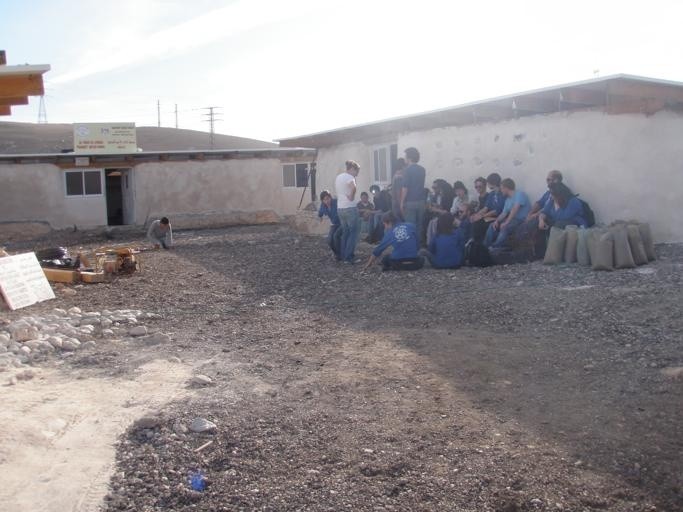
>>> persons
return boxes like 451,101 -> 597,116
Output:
316,169 -> 594,272
334,159 -> 361,265
398,148 -> 428,255
145,216 -> 172,250
390,157 -> 405,224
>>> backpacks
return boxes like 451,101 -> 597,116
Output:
580,200 -> 595,228
